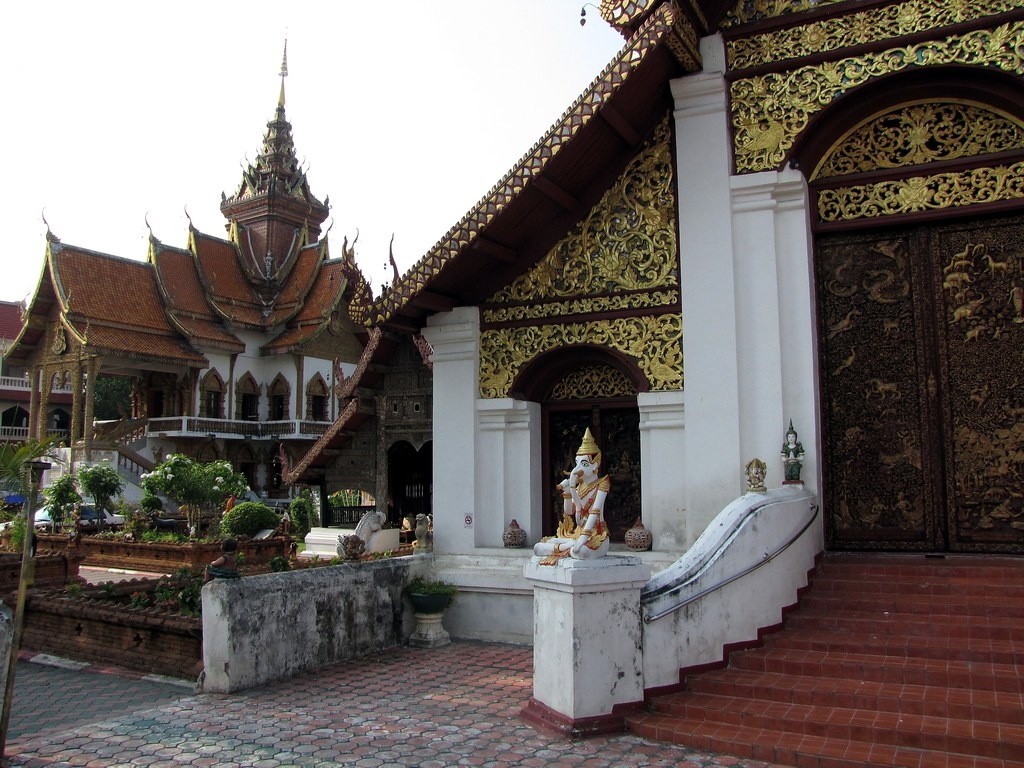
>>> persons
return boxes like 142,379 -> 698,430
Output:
780,418 -> 805,479
746,463 -> 765,488
534,429 -> 610,559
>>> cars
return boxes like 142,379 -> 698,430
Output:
0,501 -> 129,536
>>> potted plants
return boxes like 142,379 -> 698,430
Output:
402,575 -> 458,613
